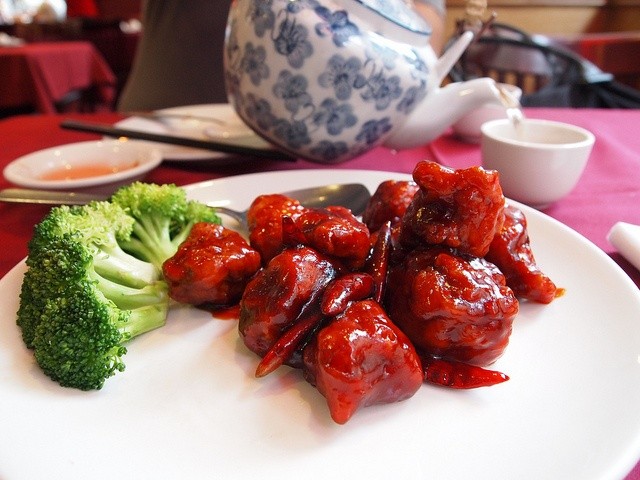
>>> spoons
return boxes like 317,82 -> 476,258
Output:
0,182 -> 370,229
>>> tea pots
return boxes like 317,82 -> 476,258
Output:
223,0 -> 512,163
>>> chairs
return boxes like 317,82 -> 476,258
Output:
440,37 -> 588,114
455,19 -> 533,49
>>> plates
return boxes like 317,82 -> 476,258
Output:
0,168 -> 640,480
116,103 -> 273,161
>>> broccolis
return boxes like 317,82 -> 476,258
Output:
24,198 -> 159,286
110,181 -> 221,273
16,237 -> 166,390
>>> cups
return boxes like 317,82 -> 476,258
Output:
481,116 -> 595,212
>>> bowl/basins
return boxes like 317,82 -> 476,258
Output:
4,138 -> 161,187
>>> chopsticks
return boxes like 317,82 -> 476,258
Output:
57,121 -> 296,162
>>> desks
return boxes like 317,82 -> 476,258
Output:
0,40 -> 117,114
